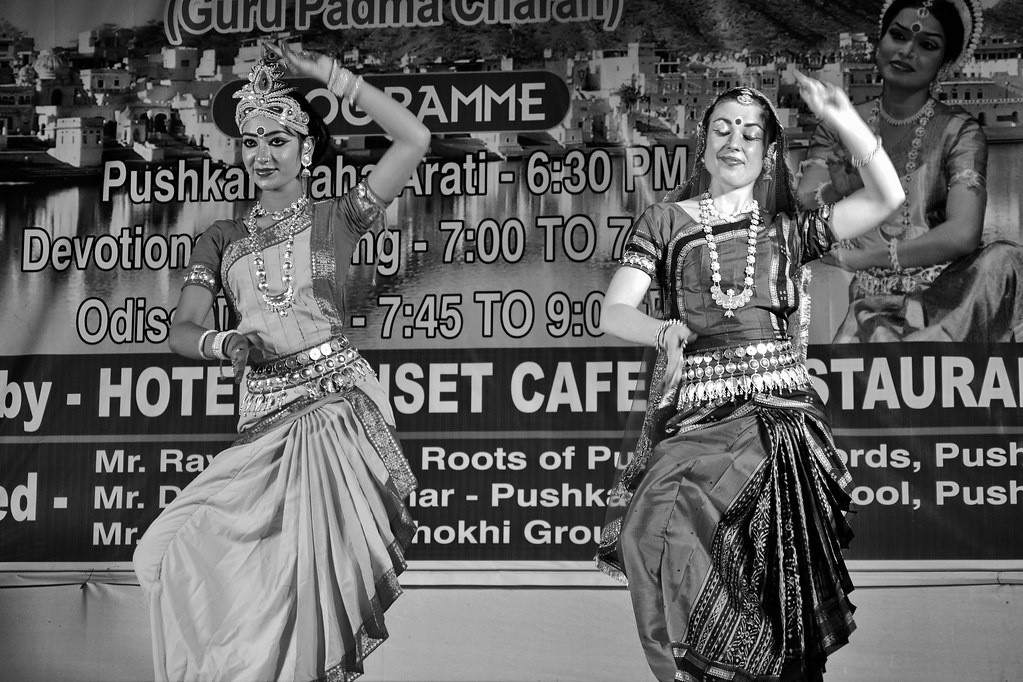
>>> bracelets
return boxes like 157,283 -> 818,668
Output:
889,236 -> 903,272
655,319 -> 691,356
198,329 -> 244,366
852,135 -> 883,169
328,59 -> 363,107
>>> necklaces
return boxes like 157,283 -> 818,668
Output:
248,194 -> 308,317
868,97 -> 934,229
699,191 -> 759,318
879,99 -> 931,125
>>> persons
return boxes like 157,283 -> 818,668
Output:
133,42 -> 431,682
795,0 -> 1023,343
595,69 -> 907,682
